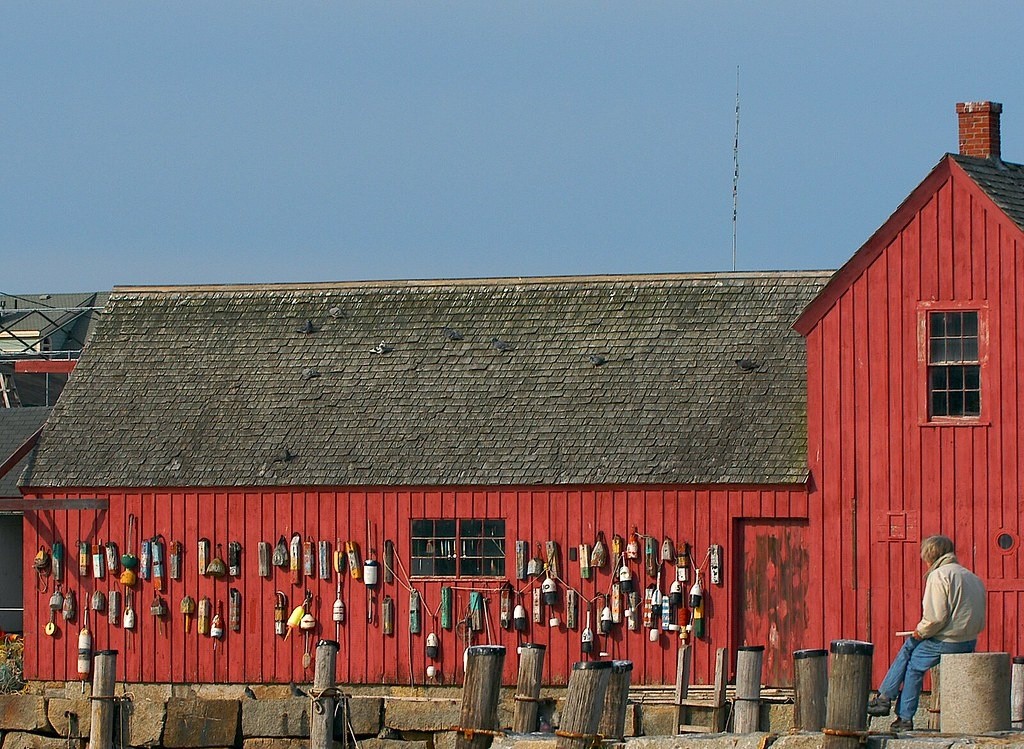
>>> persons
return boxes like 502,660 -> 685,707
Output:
867,535 -> 987,729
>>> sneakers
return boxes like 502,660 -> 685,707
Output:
868,697 -> 891,717
890,716 -> 913,732
